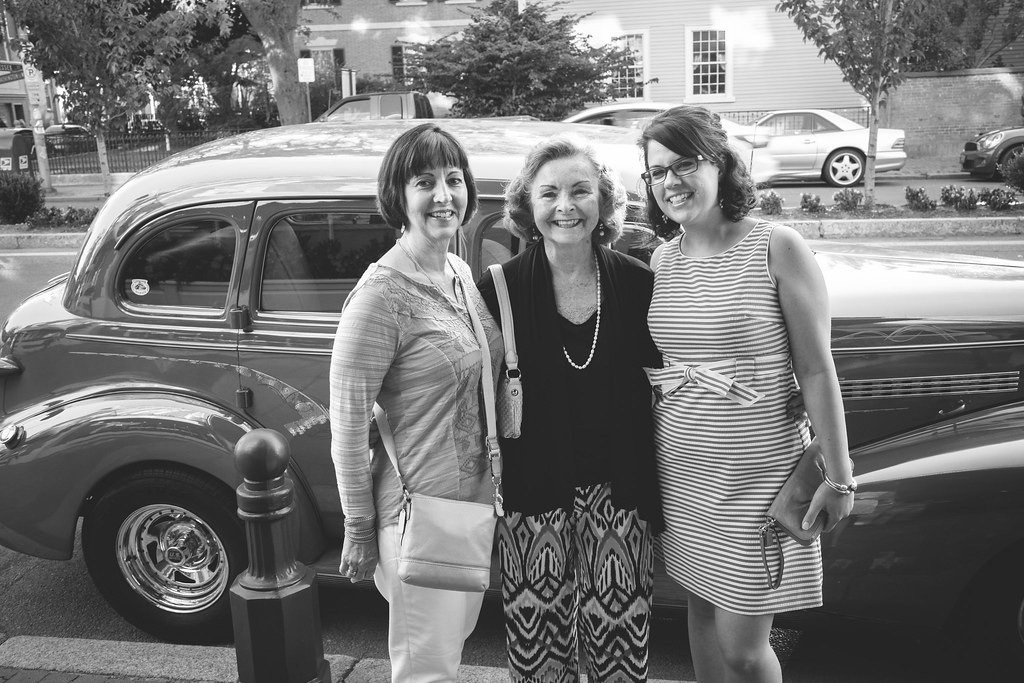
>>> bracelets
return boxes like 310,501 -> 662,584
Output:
344,527 -> 376,543
824,472 -> 857,495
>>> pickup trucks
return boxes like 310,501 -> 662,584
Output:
311,89 -> 542,123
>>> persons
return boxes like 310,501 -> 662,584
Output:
642,105 -> 858,683
329,123 -> 504,683
476,138 -> 665,683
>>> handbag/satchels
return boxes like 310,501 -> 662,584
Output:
487,263 -> 523,438
398,493 -> 498,593
758,436 -> 855,590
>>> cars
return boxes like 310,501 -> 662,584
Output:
552,100 -> 779,192
958,125 -> 1024,185
0,114 -> 1024,683
1,119 -> 171,162
739,108 -> 908,188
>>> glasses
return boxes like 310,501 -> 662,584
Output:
642,153 -> 714,186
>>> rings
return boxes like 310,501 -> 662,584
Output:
347,569 -> 357,575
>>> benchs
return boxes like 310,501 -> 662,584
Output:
143,220 -> 320,311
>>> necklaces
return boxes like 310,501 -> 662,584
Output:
397,239 -> 483,347
562,249 -> 601,370
